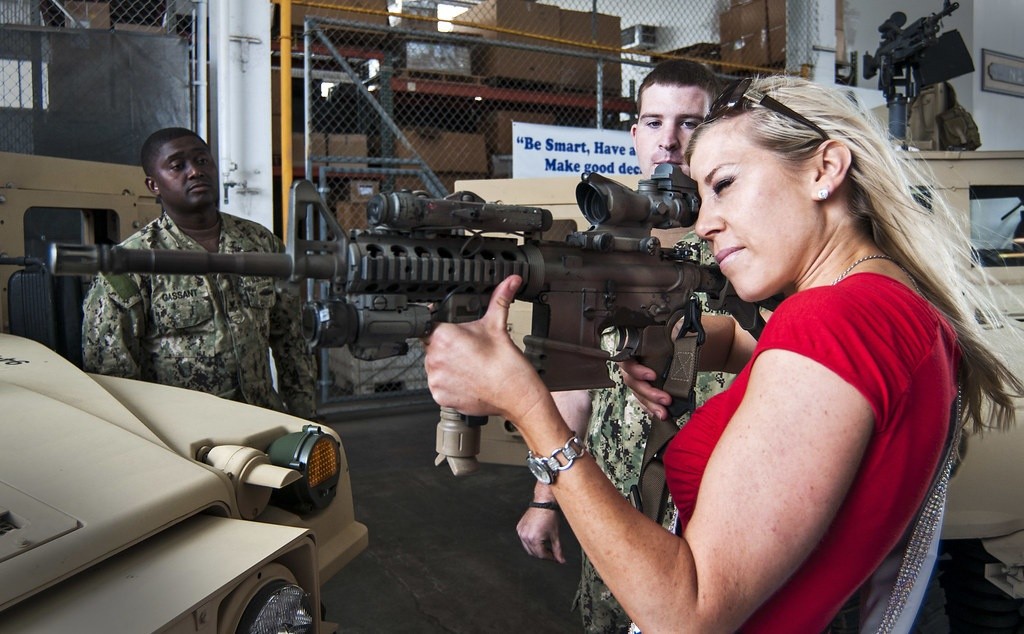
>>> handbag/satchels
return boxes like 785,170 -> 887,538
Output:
936,105 -> 981,152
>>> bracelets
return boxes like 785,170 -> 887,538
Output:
526,498 -> 561,512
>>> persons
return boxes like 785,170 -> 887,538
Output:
82,128 -> 322,428
516,58 -> 779,634
424,75 -> 1024,634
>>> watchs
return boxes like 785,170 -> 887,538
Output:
525,430 -> 586,485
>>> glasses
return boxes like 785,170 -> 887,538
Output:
703,77 -> 830,141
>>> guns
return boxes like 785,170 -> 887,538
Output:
862,0 -> 961,100
46,164 -> 784,421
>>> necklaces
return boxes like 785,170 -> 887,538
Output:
828,254 -> 938,298
